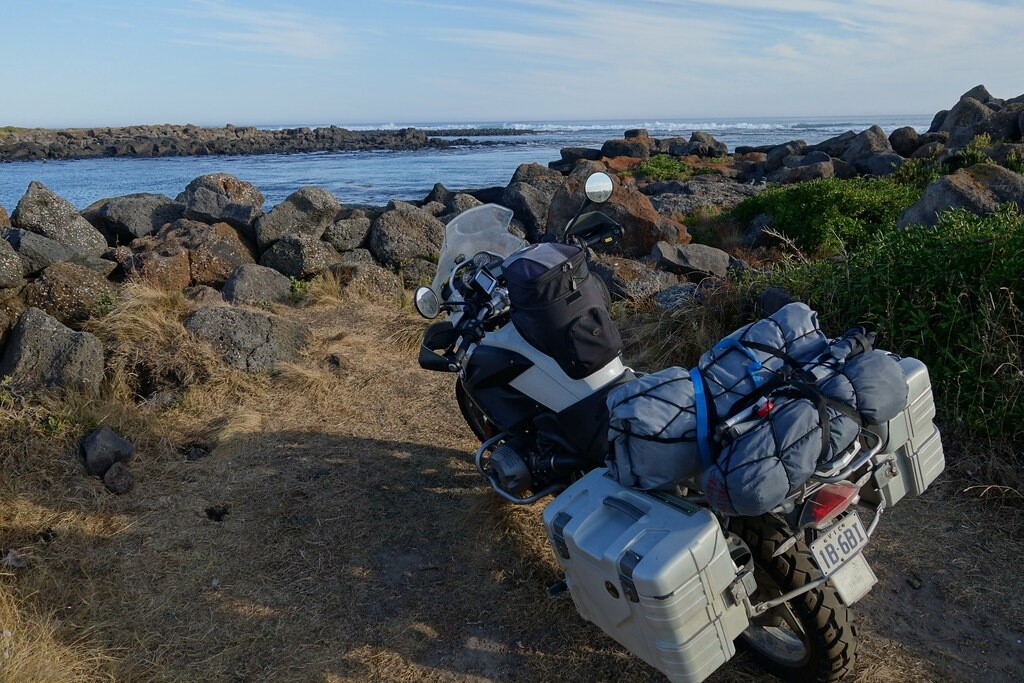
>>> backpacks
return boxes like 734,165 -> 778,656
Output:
503,242 -> 623,380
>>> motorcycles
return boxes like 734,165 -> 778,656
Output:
415,172 -> 946,683
